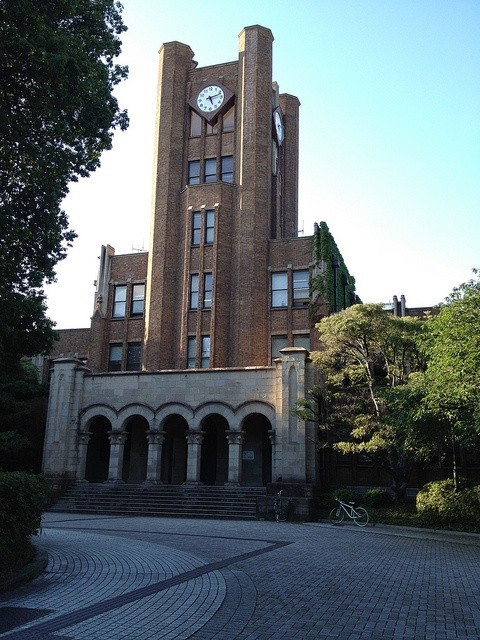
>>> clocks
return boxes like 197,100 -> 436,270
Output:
272,105 -> 287,149
188,78 -> 237,124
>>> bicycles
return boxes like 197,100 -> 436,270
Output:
329,495 -> 369,527
271,489 -> 288,523
